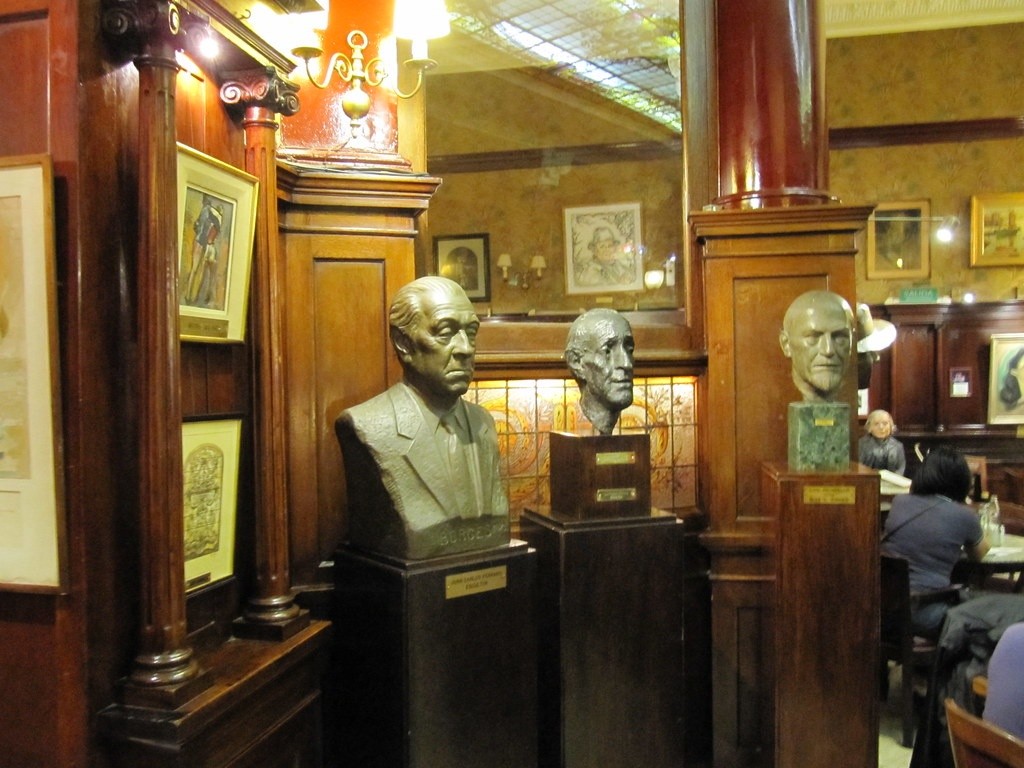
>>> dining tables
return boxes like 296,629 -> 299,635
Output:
954,534 -> 1024,594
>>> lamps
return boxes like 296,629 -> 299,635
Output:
497,254 -> 546,289
290,0 -> 450,138
644,269 -> 665,291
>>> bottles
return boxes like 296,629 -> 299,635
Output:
986,494 -> 1002,548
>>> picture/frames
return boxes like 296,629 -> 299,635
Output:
986,333 -> 1024,424
562,201 -> 645,296
949,367 -> 972,398
1,153 -> 69,595
176,141 -> 260,343
432,233 -> 491,303
182,411 -> 246,599
865,197 -> 932,280
970,191 -> 1024,266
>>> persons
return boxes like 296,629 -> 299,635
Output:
333,276 -> 510,560
777,290 -> 855,404
857,408 -> 907,476
562,308 -> 634,436
981,623 -> 1024,739
879,447 -> 993,716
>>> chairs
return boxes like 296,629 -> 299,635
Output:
880,555 -> 937,747
945,698 -> 1024,768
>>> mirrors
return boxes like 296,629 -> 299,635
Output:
425,0 -> 688,327
